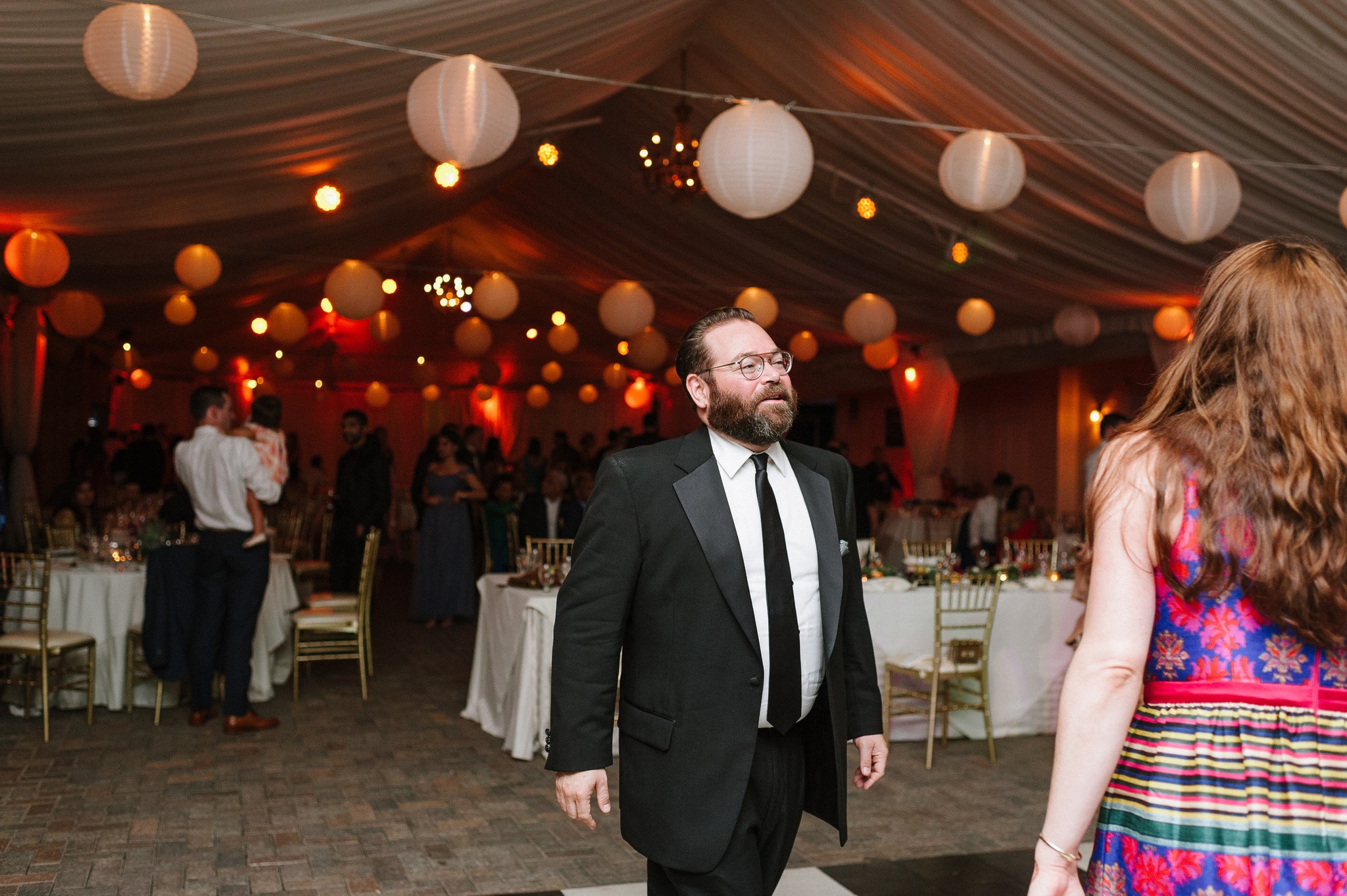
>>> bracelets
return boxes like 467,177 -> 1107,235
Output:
1038,832 -> 1083,864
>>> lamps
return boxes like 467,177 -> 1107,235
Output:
638,45 -> 708,202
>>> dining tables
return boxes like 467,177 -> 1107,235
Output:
3,551 -> 304,710
457,568 -> 1087,768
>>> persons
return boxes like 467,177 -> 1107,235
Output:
108,412 -> 1134,571
416,428 -> 488,630
172,384 -> 282,731
52,474 -> 95,546
232,395 -> 289,546
549,307 -> 886,894
1026,236 -> 1347,894
327,408 -> 391,626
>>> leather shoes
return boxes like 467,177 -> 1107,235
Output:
191,706 -> 219,726
224,710 -> 281,732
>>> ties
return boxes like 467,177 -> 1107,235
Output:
750,451 -> 803,736
996,504 -> 1006,540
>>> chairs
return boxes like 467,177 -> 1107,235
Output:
21,484 -> 171,557
0,551 -> 97,743
901,537 -> 953,559
881,570 -> 1007,769
1002,536 -> 1060,573
123,622 -> 226,726
525,535 -> 576,568
480,510 -> 523,578
258,499 -> 383,702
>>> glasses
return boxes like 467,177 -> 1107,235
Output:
693,350 -> 796,384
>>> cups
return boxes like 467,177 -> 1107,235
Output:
520,548 -> 537,573
